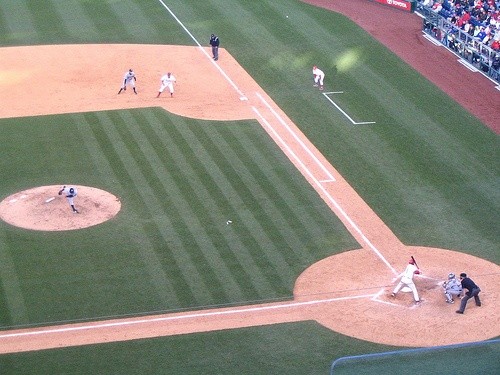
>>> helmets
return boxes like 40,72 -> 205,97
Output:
448,272 -> 454,278
70,188 -> 74,193
408,258 -> 414,264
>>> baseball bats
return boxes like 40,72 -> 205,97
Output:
411,255 -> 419,269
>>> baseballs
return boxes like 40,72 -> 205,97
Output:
227,221 -> 232,224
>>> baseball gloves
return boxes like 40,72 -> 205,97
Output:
442,281 -> 446,288
58,190 -> 62,195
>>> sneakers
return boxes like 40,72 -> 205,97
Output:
445,299 -> 454,303
456,294 -> 463,298
391,293 -> 395,297
415,301 -> 419,305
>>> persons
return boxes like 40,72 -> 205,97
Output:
156,73 -> 176,97
58,186 -> 80,214
456,273 -> 481,314
417,0 -> 500,83
209,34 -> 220,61
440,273 -> 463,303
390,258 -> 423,304
312,65 -> 325,90
117,69 -> 138,94
